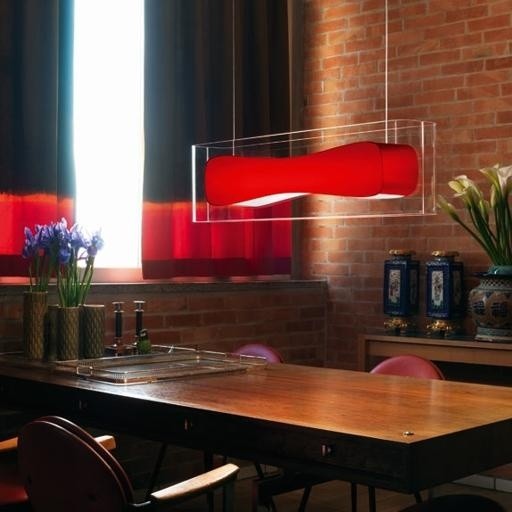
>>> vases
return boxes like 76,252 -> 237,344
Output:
24,289 -> 106,360
464,266 -> 511,341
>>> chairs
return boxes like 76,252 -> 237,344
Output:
233,343 -> 447,512
17,415 -> 241,511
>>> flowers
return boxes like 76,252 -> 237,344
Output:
436,162 -> 512,267
22,217 -> 105,307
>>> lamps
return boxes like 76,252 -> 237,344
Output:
190,1 -> 437,224
